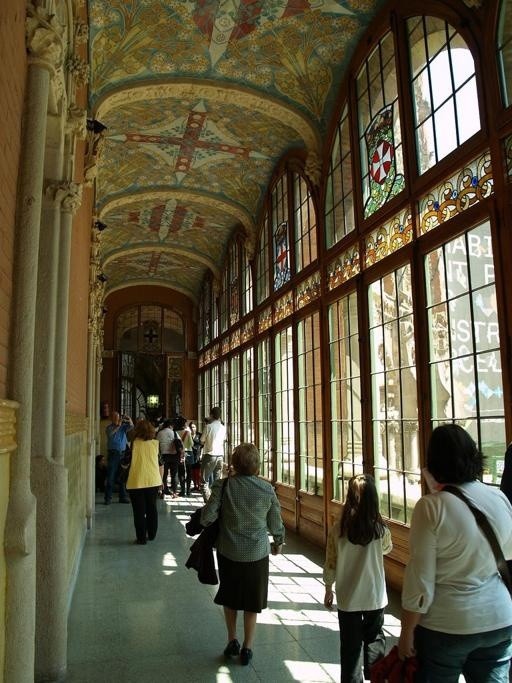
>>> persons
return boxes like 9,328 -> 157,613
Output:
122,417 -> 164,545
199,441 -> 286,667
322,474 -> 394,682
89,400 -> 227,508
396,422 -> 511,682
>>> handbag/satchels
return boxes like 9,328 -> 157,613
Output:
123,445 -> 132,465
174,439 -> 183,452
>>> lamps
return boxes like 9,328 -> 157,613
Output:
95,222 -> 108,231
86,119 -> 107,134
97,274 -> 109,282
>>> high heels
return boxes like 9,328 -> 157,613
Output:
241,649 -> 253,666
224,639 -> 240,656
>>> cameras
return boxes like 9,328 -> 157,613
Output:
121,415 -> 126,420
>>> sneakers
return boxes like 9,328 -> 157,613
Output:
158,489 -> 190,499
119,498 -> 130,504
137,534 -> 156,544
104,499 -> 112,504
